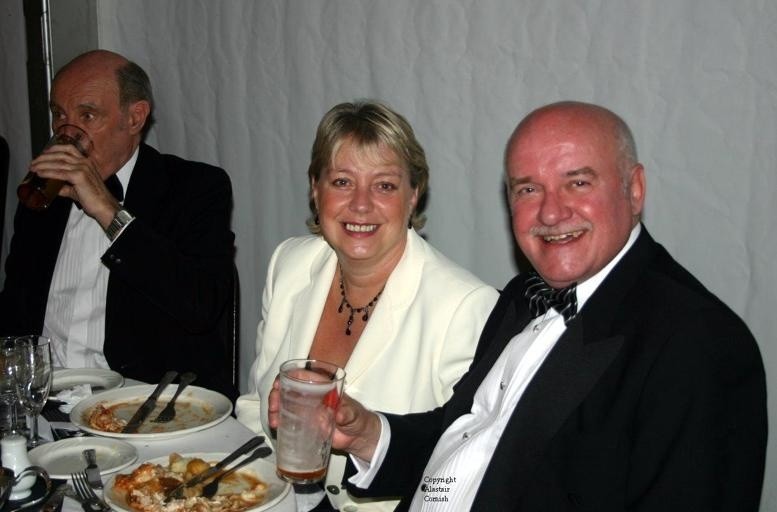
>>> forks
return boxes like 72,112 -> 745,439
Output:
68,470 -> 109,512
149,370 -> 198,422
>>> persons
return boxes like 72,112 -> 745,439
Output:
234,101 -> 500,511
0,49 -> 242,418
268,101 -> 768,512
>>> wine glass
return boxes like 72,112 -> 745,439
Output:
0,335 -> 52,446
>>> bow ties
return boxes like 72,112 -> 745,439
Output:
72,173 -> 123,209
521,269 -> 580,324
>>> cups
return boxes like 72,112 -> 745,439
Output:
16,124 -> 93,213
274,357 -> 348,486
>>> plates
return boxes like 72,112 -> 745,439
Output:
103,451 -> 291,511
48,365 -> 122,397
27,436 -> 139,480
67,383 -> 236,441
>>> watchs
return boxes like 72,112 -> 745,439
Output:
105,207 -> 133,243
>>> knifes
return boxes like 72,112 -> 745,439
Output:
153,436 -> 270,500
81,449 -> 103,490
119,370 -> 178,436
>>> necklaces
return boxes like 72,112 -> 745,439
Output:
338,263 -> 385,335
